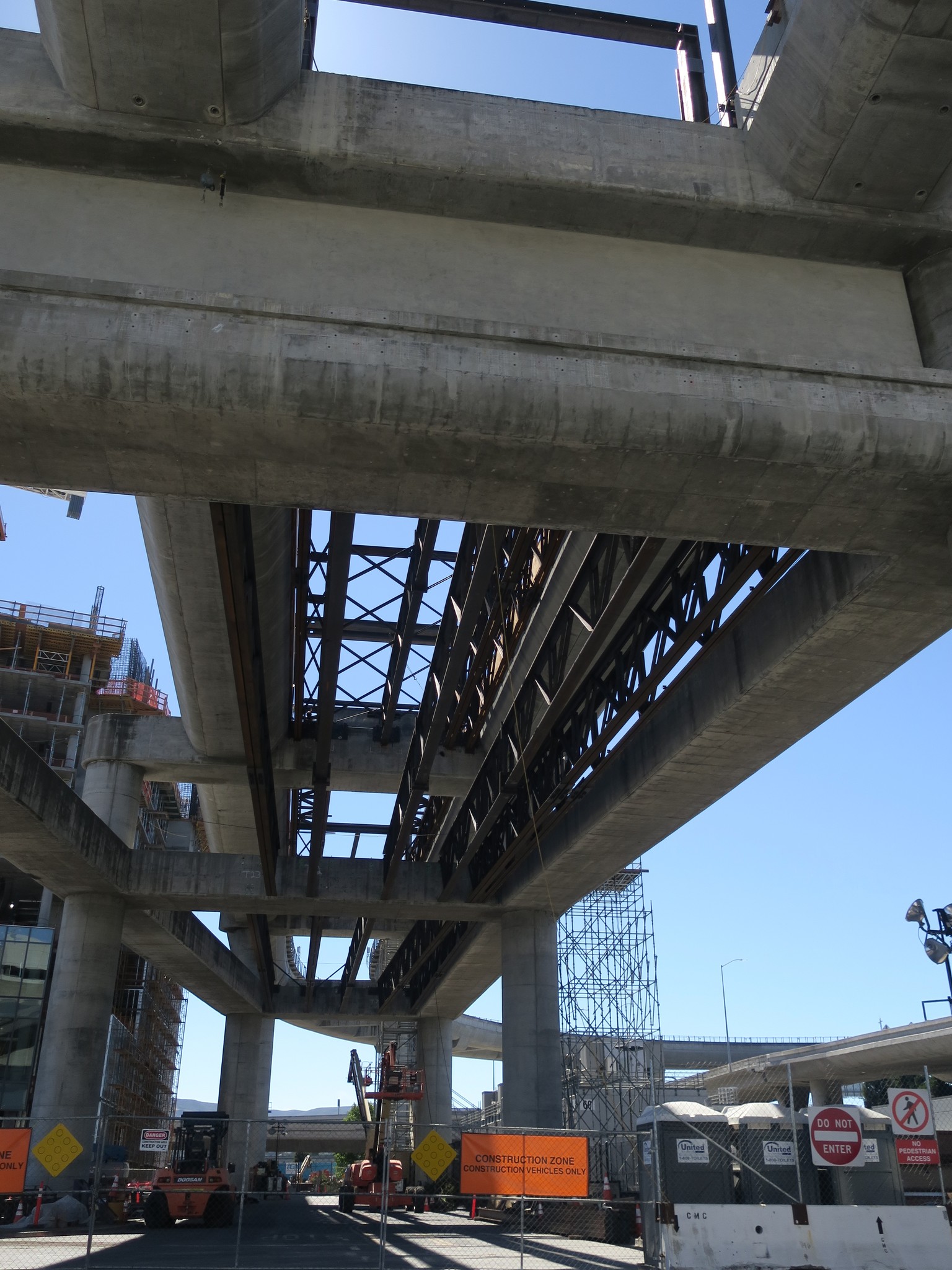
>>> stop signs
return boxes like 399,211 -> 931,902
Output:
808,1107 -> 866,1167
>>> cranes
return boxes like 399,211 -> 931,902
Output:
0,481 -> 87,544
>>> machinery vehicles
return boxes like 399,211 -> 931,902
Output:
142,1111 -> 234,1230
339,1040 -> 427,1213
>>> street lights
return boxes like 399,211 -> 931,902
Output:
904,898 -> 952,995
720,957 -> 742,1072
